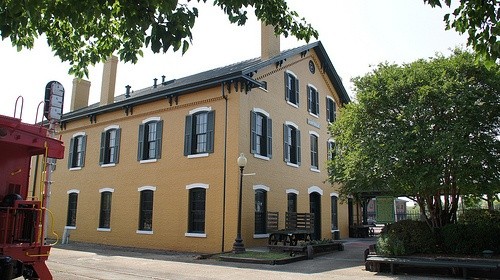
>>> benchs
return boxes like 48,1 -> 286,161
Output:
366,257 -> 500,280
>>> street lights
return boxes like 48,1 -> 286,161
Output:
231,152 -> 247,254
